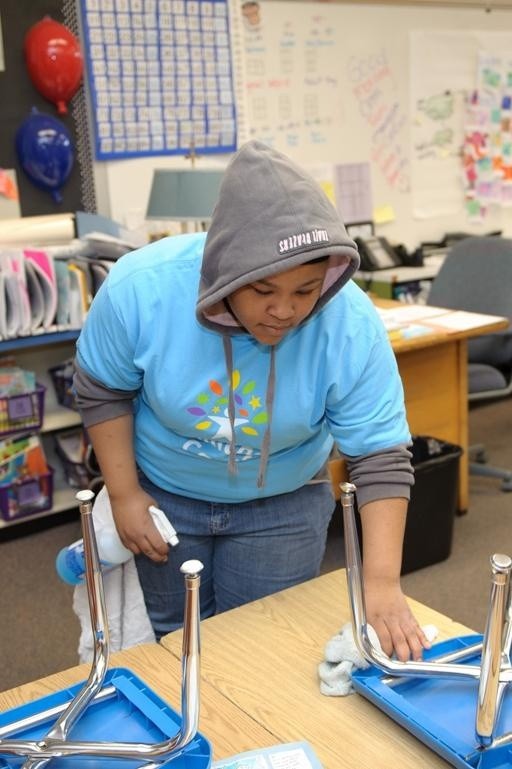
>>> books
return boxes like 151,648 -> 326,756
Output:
2,234 -> 142,338
0,356 -> 40,431
0,432 -> 50,517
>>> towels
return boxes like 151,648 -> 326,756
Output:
71,479 -> 157,662
317,622 -> 437,697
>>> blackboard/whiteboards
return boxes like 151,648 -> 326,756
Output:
71,2 -> 511,255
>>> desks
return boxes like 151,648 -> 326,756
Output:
160,564 -> 510,769
349,255 -> 437,303
1,640 -> 320,769
327,288 -> 511,523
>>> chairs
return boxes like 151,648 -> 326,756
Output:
427,237 -> 512,494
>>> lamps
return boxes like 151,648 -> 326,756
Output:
144,165 -> 222,237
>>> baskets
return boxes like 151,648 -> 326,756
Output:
55,430 -> 97,489
48,363 -> 80,412
0,458 -> 56,521
1,381 -> 47,436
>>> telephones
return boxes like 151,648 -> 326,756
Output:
355,235 -> 400,271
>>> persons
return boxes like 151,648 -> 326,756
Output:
73,140 -> 431,666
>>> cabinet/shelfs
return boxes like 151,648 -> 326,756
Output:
0,254 -> 114,529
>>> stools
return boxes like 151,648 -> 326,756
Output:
1,487 -> 211,768
336,471 -> 511,769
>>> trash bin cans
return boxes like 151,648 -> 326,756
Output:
402,435 -> 464,577
